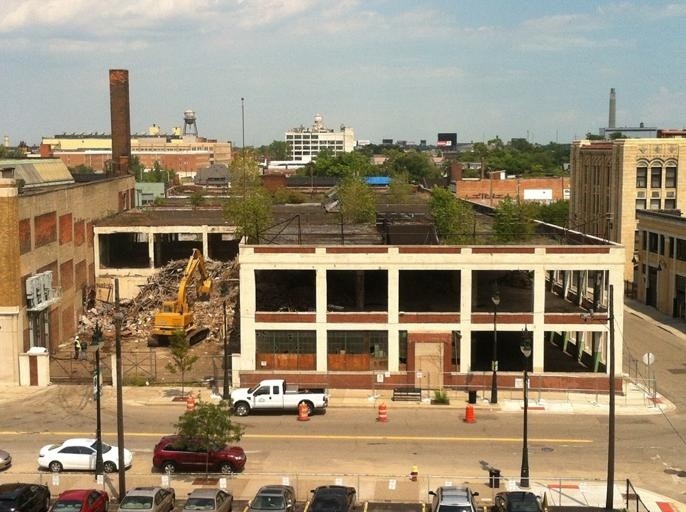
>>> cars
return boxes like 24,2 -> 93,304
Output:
522,504 -> 527,506
117,486 -> 175,512
245,484 -> 297,512
494,492 -> 543,512
0,483 -> 51,512
181,488 -> 232,512
429,486 -> 479,512
0,449 -> 11,471
49,489 -> 109,512
305,485 -> 356,512
37,438 -> 132,474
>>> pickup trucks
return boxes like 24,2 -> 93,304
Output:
230,379 -> 328,417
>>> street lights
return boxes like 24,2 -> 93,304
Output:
88,321 -> 104,480
488,291 -> 501,402
515,319 -> 533,485
221,300 -> 236,402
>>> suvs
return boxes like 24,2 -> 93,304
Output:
152,434 -> 246,475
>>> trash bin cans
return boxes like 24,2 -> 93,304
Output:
468,390 -> 477,404
490,469 -> 501,488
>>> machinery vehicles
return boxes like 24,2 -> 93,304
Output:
145,247 -> 214,347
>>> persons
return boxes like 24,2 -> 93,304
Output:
73,336 -> 81,360
